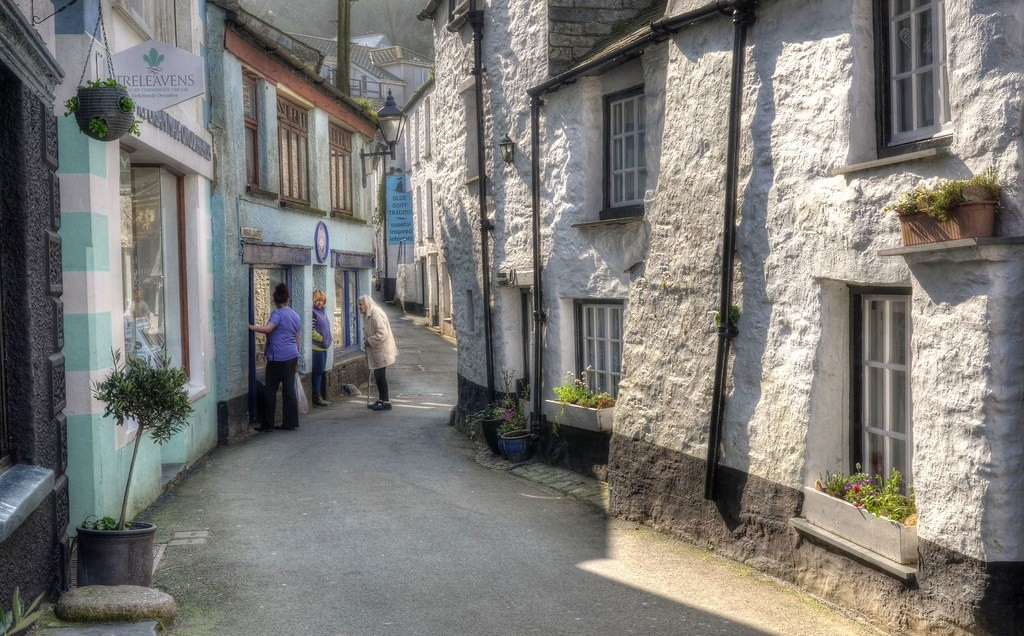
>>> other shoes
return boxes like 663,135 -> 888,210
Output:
314,395 -> 332,406
274,424 -> 295,430
254,426 -> 273,432
368,401 -> 392,410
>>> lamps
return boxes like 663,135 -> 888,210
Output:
497,134 -> 516,165
375,87 -> 408,160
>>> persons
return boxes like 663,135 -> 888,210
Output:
312,289 -> 333,402
357,294 -> 399,410
248,283 -> 302,430
128,287 -> 150,321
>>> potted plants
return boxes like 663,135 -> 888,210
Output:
501,379 -> 536,464
62,78 -> 143,143
882,172 -> 1002,248
75,343 -> 194,588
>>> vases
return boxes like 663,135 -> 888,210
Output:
545,399 -> 616,433
481,417 -> 508,456
804,485 -> 919,566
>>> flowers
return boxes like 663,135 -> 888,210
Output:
814,464 -> 919,528
465,368 -> 517,439
552,365 -> 617,439
